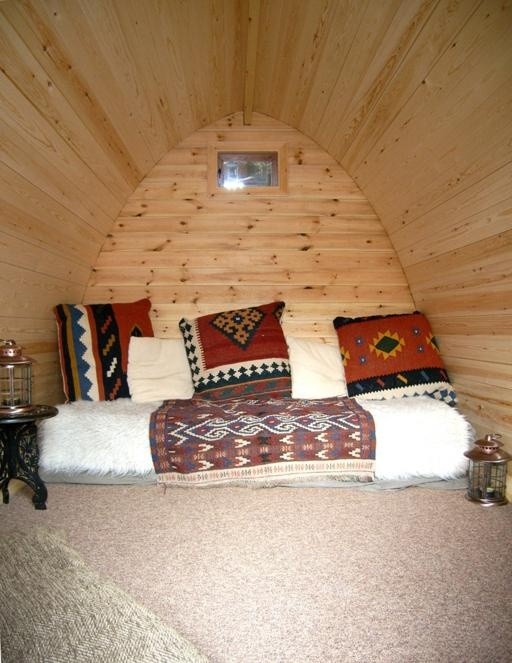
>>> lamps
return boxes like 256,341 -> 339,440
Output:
464,433 -> 511,506
0,339 -> 36,416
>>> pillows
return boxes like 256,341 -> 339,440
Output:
179,300 -> 293,400
287,338 -> 350,399
127,336 -> 197,402
55,298 -> 154,402
333,310 -> 456,403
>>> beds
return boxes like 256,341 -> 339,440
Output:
34,398 -> 474,489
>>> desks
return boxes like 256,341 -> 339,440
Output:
0,405 -> 59,511
